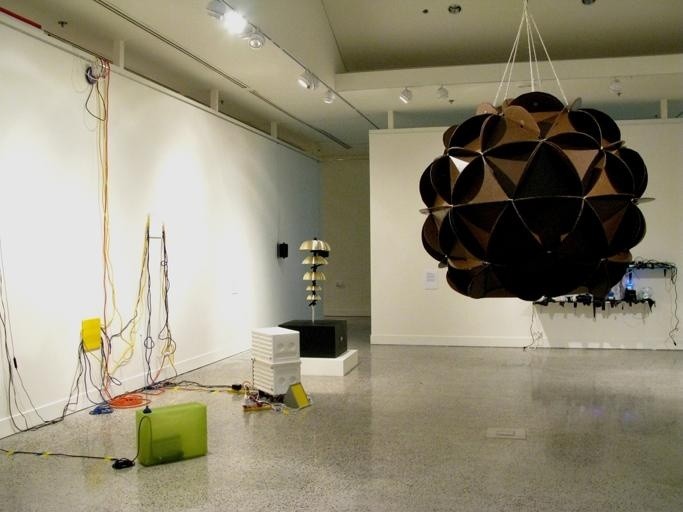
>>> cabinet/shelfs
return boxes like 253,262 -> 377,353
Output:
535,266 -> 674,318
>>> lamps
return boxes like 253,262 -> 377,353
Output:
206,0 -> 334,104
400,87 -> 413,103
436,85 -> 448,100
